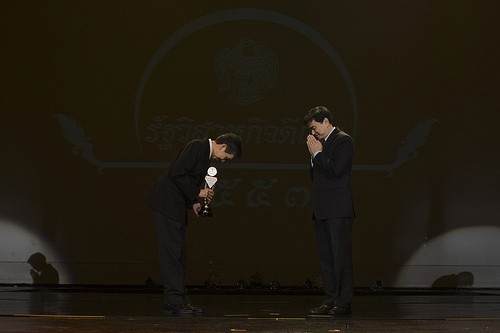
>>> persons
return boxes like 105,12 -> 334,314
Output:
304,106 -> 356,315
144,133 -> 242,314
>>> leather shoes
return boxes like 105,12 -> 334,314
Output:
310,305 -> 329,314
328,304 -> 352,315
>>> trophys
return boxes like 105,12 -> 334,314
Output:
197,167 -> 217,216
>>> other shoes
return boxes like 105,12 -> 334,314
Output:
184,305 -> 205,313
165,305 -> 195,314
